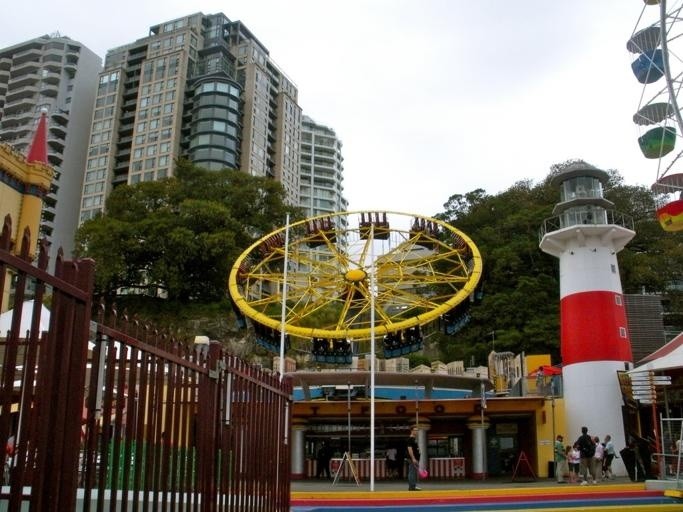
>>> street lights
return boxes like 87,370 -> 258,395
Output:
554,446 -> 565,453
419,468 -> 429,479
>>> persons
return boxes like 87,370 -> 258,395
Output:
385,445 -> 397,475
549,378 -> 554,396
314,441 -> 333,480
551,373 -> 559,396
675,439 -> 683,455
662,430 -> 676,477
534,365 -> 544,396
406,425 -> 420,491
552,426 -> 620,486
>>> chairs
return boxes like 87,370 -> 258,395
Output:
409,486 -> 421,491
557,475 -> 614,485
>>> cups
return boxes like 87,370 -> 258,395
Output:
413,430 -> 419,431
